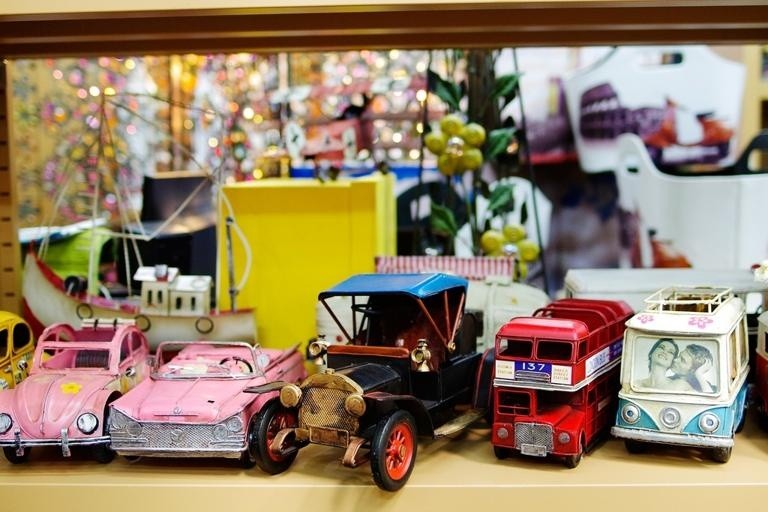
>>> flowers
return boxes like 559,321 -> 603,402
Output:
407,48 -> 539,283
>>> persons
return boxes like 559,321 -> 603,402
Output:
634,338 -> 718,394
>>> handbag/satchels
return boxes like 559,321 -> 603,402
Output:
562,45 -> 745,175
